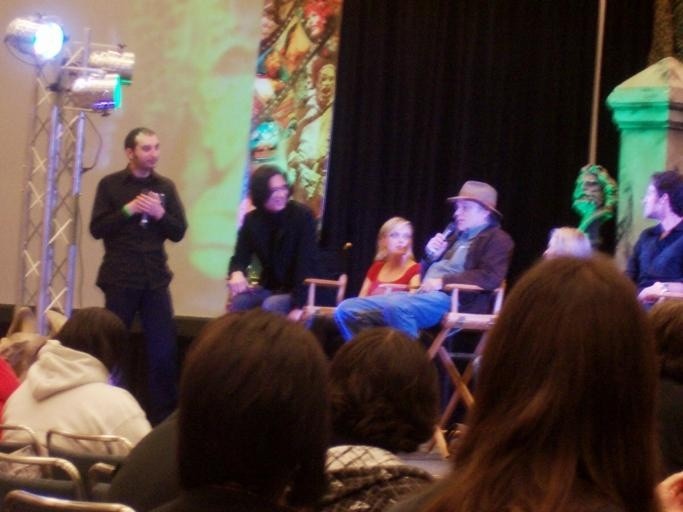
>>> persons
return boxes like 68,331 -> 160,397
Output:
624,169 -> 683,292
227,165 -> 319,315
90,128 -> 187,423
562,162 -> 618,254
544,225 -> 595,261
333,179 -> 515,343
238,1 -> 341,225
2,255 -> 682,511
358,217 -> 422,299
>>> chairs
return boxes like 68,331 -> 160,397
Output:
226,273 -> 348,320
378,280 -> 506,458
616,264 -> 682,310
0,425 -> 136,512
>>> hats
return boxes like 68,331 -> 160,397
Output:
448,180 -> 503,216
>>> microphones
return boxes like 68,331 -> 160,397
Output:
425,222 -> 457,260
138,187 -> 153,227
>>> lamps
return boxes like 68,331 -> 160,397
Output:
3,14 -> 136,117
